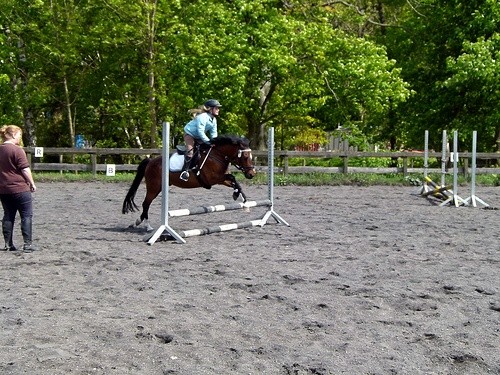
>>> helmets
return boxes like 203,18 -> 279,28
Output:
204,99 -> 223,107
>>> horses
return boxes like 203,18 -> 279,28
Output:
120,132 -> 256,232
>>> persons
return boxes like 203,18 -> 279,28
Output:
0,125 -> 38,251
182,99 -> 222,179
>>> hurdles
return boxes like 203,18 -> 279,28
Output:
410,130 -> 469,208
440,130 -> 490,208
143,121 -> 289,246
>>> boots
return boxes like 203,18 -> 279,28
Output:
21,215 -> 32,252
181,156 -> 193,178
2,216 -> 17,251
